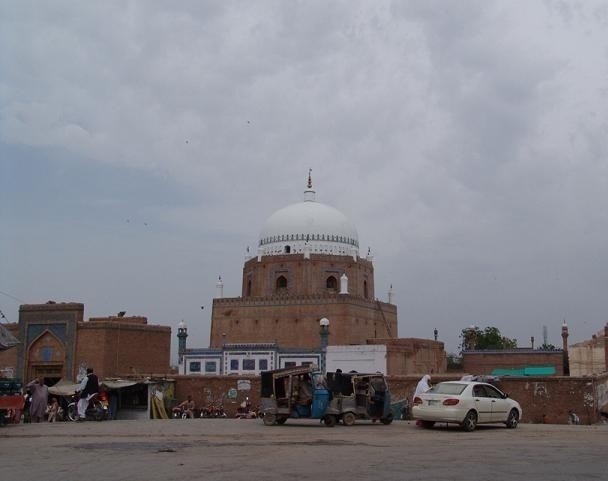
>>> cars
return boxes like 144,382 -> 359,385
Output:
412,381 -> 522,432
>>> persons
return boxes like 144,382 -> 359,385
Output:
172,394 -> 198,420
48,398 -> 59,423
566,410 -> 581,425
25,376 -> 49,424
74,367 -> 99,422
412,374 -> 432,427
358,378 -> 380,417
457,376 -> 476,398
298,373 -> 313,401
542,415 -> 550,424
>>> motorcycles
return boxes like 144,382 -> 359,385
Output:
178,401 -> 226,419
67,390 -> 108,422
259,364 -> 394,427
